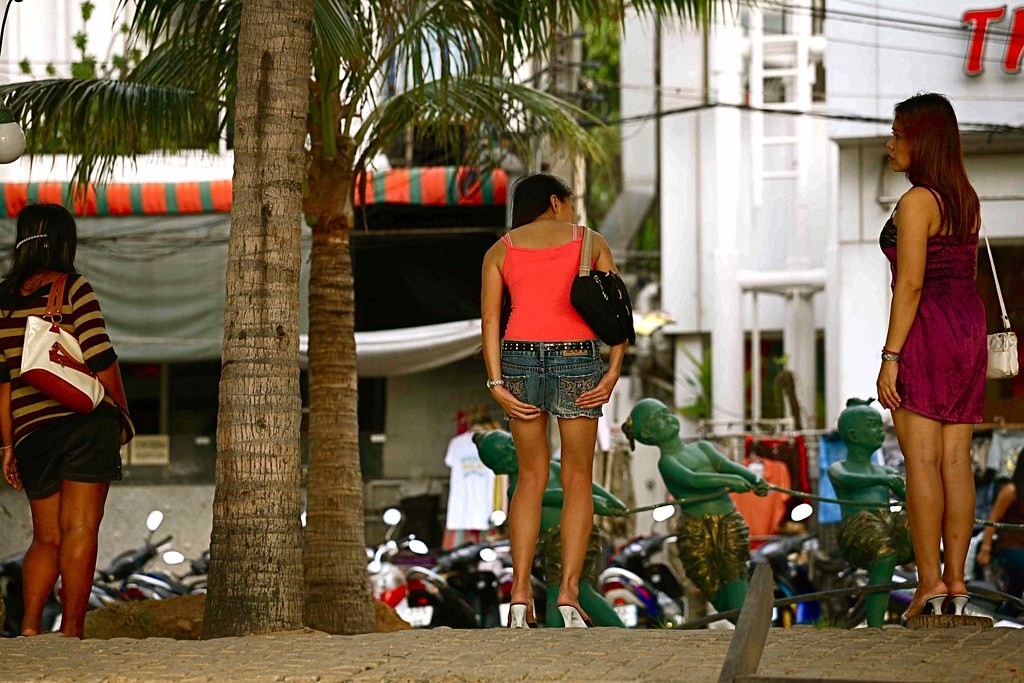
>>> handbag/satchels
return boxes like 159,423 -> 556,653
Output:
570,227 -> 636,346
19,274 -> 105,412
986,332 -> 1019,379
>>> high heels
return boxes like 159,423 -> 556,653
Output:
943,594 -> 970,615
901,593 -> 947,625
558,605 -> 594,628
509,604 -> 538,629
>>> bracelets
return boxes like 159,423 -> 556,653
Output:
981,544 -> 990,552
882,346 -> 900,356
0,444 -> 12,455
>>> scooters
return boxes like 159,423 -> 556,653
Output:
597,505 -> 737,630
364,501 -> 547,635
745,498 -> 1024,628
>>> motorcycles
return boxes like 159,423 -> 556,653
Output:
0,509 -> 212,636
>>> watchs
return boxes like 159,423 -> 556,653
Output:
487,379 -> 505,390
881,353 -> 898,361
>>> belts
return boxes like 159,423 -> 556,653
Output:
503,339 -> 601,352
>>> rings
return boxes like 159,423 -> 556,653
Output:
10,474 -> 14,476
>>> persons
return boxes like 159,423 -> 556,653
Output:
977,447 -> 1024,618
479,175 -> 628,631
621,397 -> 768,627
875,92 -> 987,620
0,202 -> 136,641
475,427 -> 627,629
828,398 -> 914,630
